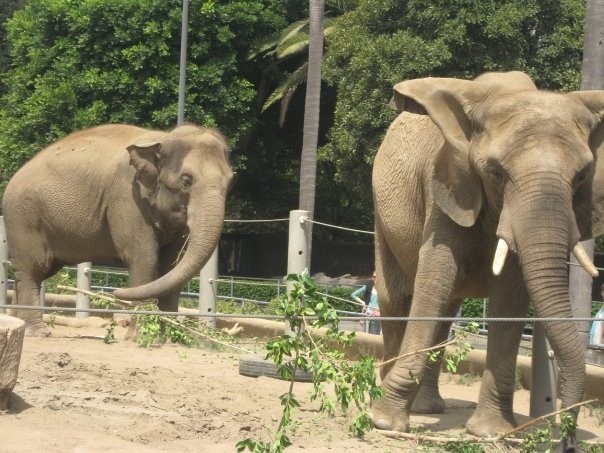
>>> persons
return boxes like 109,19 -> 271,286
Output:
350,268 -> 380,335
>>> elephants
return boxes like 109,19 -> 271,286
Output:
1,123 -> 238,337
366,71 -> 604,453
